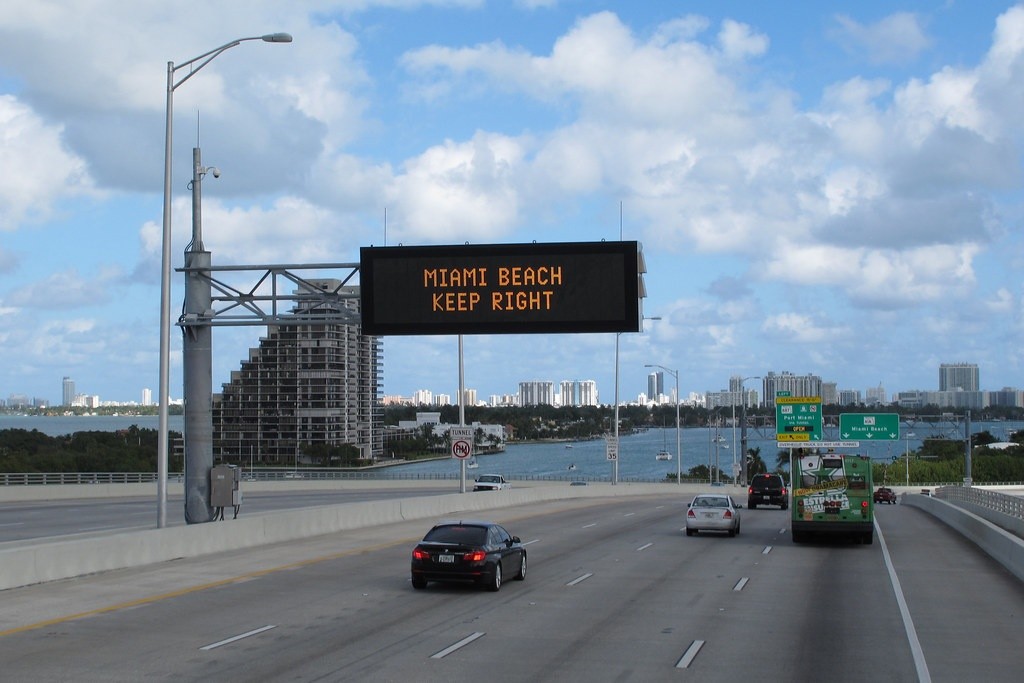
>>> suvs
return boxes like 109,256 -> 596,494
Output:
746,472 -> 791,511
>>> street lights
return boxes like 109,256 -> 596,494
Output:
611,316 -> 661,483
645,364 -> 680,486
732,376 -> 762,487
157,31 -> 294,527
906,431 -> 916,486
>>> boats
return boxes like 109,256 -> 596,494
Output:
467,452 -> 478,469
569,464 -> 576,471
712,432 -> 730,449
565,440 -> 574,449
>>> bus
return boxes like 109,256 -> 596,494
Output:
789,448 -> 874,545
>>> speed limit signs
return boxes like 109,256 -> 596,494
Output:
606,442 -> 618,460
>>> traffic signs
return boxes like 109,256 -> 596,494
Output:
774,396 -> 823,442
838,413 -> 900,442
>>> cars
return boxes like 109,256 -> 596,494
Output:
411,520 -> 527,592
685,493 -> 742,538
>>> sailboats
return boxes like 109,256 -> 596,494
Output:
656,415 -> 673,461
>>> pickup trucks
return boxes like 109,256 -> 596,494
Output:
473,474 -> 512,492
873,487 -> 897,504
